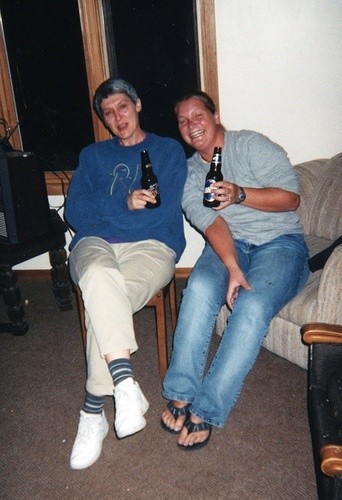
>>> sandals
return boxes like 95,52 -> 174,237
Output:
160,399 -> 192,434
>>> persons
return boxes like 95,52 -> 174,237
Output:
63,78 -> 189,470
160,89 -> 311,451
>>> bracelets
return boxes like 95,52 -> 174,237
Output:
235,185 -> 247,204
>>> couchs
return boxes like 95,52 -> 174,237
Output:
215,153 -> 342,369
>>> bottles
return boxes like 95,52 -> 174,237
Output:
140,150 -> 161,209
203,147 -> 223,208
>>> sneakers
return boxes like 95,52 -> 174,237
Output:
71,409 -> 109,470
114,376 -> 149,438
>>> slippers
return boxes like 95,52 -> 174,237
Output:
177,413 -> 212,451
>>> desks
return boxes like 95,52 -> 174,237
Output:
0,209 -> 72,335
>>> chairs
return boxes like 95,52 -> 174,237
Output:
74,272 -> 178,380
301,323 -> 342,500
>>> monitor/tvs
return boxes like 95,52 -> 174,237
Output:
0,150 -> 52,244
